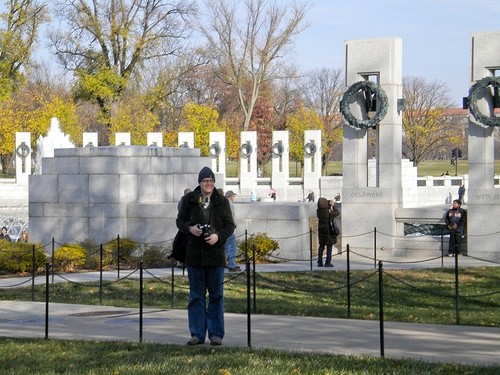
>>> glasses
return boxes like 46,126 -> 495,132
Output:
201,180 -> 214,184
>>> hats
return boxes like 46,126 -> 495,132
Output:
198,167 -> 215,184
226,191 -> 237,197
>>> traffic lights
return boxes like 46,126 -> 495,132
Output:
451,149 -> 455,158
458,149 -> 462,158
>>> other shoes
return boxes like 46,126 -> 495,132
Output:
187,336 -> 204,345
318,263 -> 323,266
325,263 -> 334,267
448,253 -> 455,257
229,267 -> 241,272
211,335 -> 222,344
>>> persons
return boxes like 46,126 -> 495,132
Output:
0,226 -> 12,244
445,199 -> 467,257
17,231 -> 30,243
171,166 -> 236,345
166,187 -> 195,270
459,184 -> 465,204
224,191 -> 243,273
305,190 -> 317,203
314,197 -> 342,267
440,170 -> 450,176
333,191 -> 343,203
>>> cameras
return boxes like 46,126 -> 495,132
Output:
199,224 -> 212,238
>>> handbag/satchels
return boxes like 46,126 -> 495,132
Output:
171,231 -> 186,262
332,226 -> 339,236
